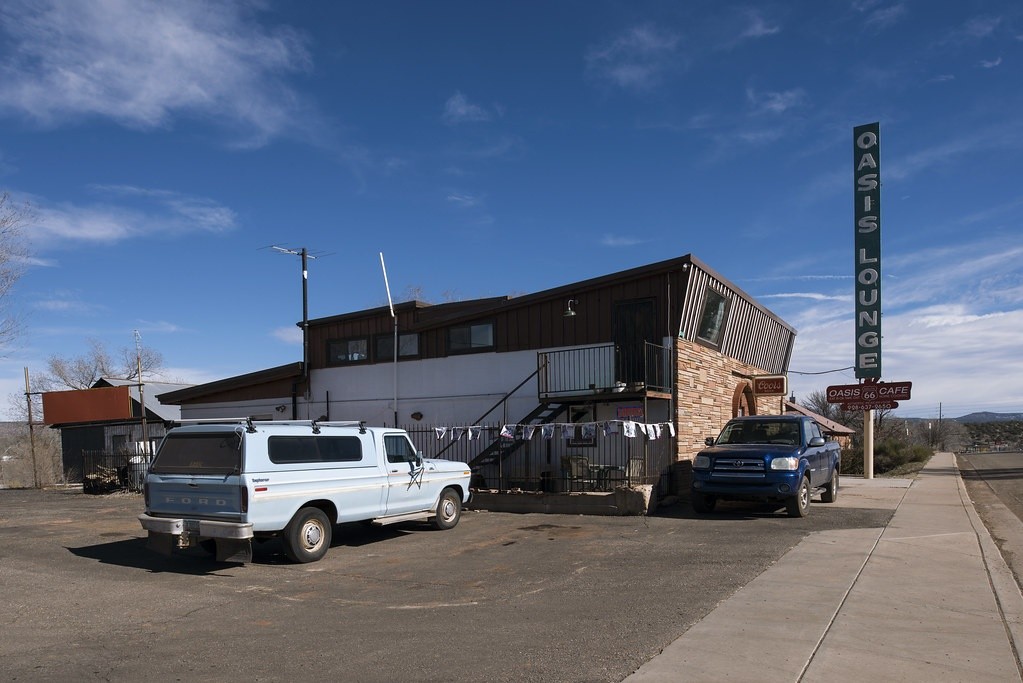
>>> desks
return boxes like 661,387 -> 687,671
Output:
590,464 -> 623,491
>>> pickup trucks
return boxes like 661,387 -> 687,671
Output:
692,415 -> 842,519
138,415 -> 473,563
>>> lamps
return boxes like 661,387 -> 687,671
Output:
561,298 -> 579,318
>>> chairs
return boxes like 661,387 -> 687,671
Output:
562,454 -> 642,492
